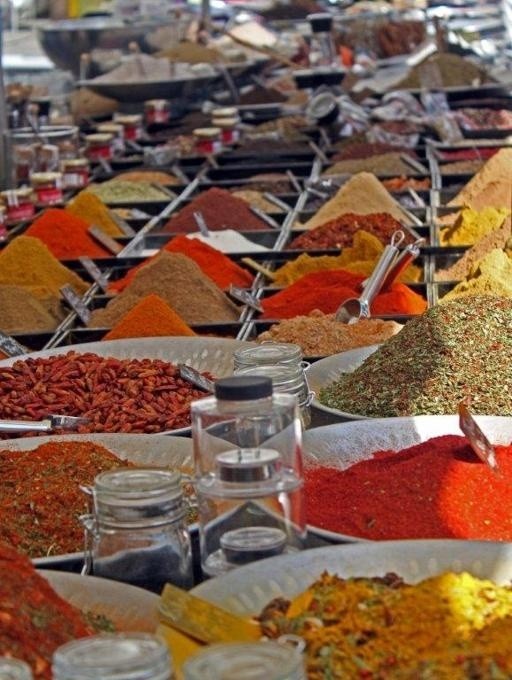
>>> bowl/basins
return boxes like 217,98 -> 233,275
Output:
293,69 -> 346,88
370,54 -> 504,100
29,16 -> 273,114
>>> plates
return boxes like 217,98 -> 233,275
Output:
250,411 -> 512,546
3,434 -> 249,564
160,535 -> 510,680
0,569 -> 158,679
2,339 -> 258,437
307,344 -> 390,420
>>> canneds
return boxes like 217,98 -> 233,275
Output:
0,99 -> 170,246
210,108 -> 241,148
192,126 -> 222,156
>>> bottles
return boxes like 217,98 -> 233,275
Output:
0,99 -> 241,241
186,338 -> 311,572
71,469 -> 190,590
308,15 -> 336,69
